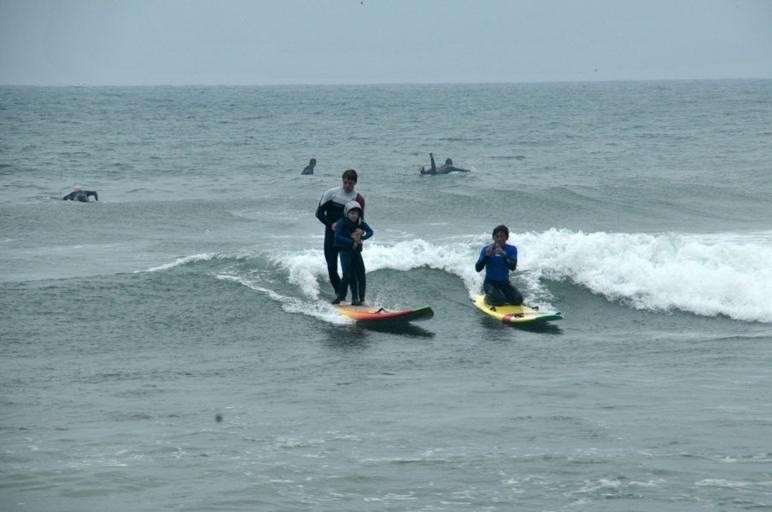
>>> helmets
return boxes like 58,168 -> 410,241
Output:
344,200 -> 362,218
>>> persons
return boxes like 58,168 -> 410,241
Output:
419,151 -> 472,175
314,169 -> 365,304
475,224 -> 524,307
334,200 -> 374,305
63,185 -> 99,202
300,158 -> 317,175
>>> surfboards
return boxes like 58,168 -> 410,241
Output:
472,295 -> 563,323
335,302 -> 434,324
